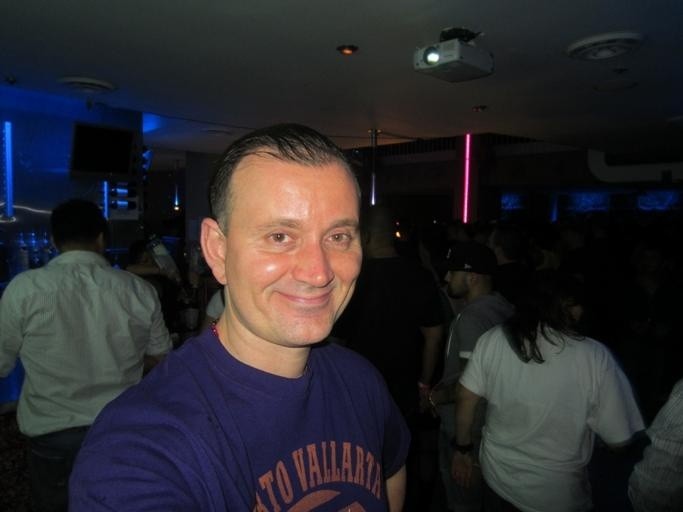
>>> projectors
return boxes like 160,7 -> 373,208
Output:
413,36 -> 496,85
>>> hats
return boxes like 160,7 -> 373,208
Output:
434,241 -> 496,275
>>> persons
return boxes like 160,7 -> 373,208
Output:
324,202 -> 683,511
0,199 -> 174,512
63,123 -> 416,510
122,218 -> 225,337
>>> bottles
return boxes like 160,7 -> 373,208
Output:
149,240 -> 179,281
2,227 -> 57,274
96,185 -> 137,211
